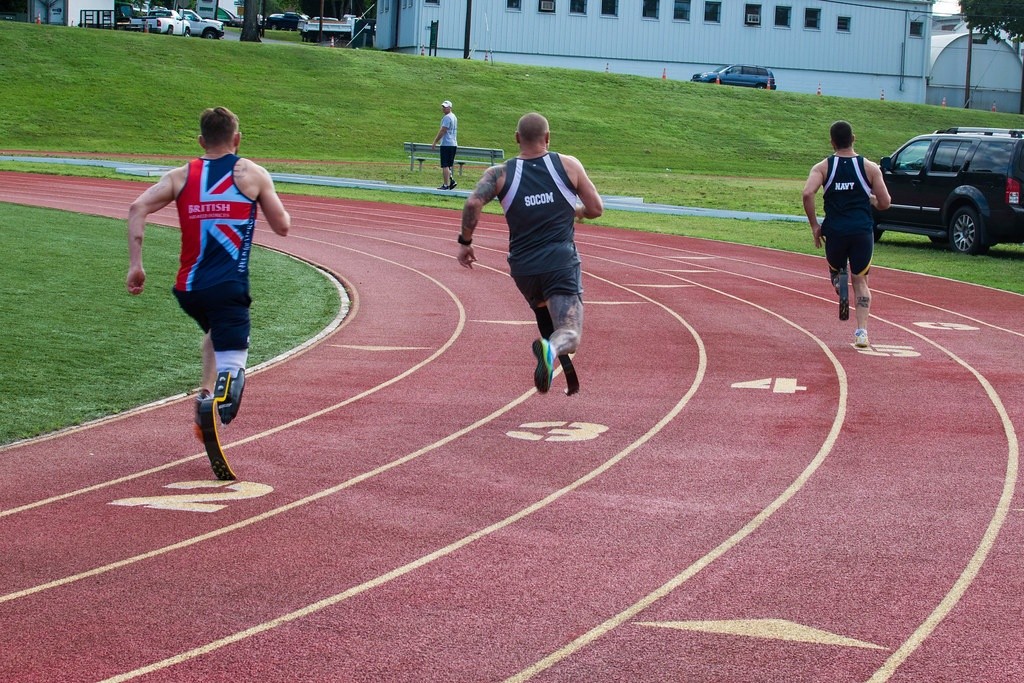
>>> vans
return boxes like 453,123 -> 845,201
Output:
690,63 -> 776,90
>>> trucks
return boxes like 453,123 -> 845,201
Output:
298,15 -> 377,42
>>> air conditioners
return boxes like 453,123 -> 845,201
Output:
746,14 -> 759,23
541,1 -> 554,10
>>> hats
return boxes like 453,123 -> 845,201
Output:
441,100 -> 452,107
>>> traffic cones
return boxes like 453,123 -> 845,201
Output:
942,97 -> 946,107
420,45 -> 425,56
816,82 -> 822,96
36,10 -> 40,24
715,73 -> 719,85
766,78 -> 770,89
880,89 -> 885,100
605,63 -> 609,72
485,49 -> 489,61
329,36 -> 334,48
662,68 -> 666,79
992,102 -> 996,112
144,21 -> 148,33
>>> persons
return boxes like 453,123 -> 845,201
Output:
126,106 -> 290,446
301,22 -> 309,42
802,119 -> 891,348
455,112 -> 604,394
431,100 -> 458,190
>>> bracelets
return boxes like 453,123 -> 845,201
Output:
457,234 -> 473,246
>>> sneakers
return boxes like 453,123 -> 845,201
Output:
854,331 -> 869,348
195,389 -> 210,445
449,180 -> 457,190
437,184 -> 449,190
532,336 -> 555,394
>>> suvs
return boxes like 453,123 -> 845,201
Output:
873,126 -> 1024,255
216,7 -> 338,32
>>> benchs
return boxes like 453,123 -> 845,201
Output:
403,141 -> 504,176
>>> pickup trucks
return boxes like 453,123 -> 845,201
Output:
126,5 -> 224,40
